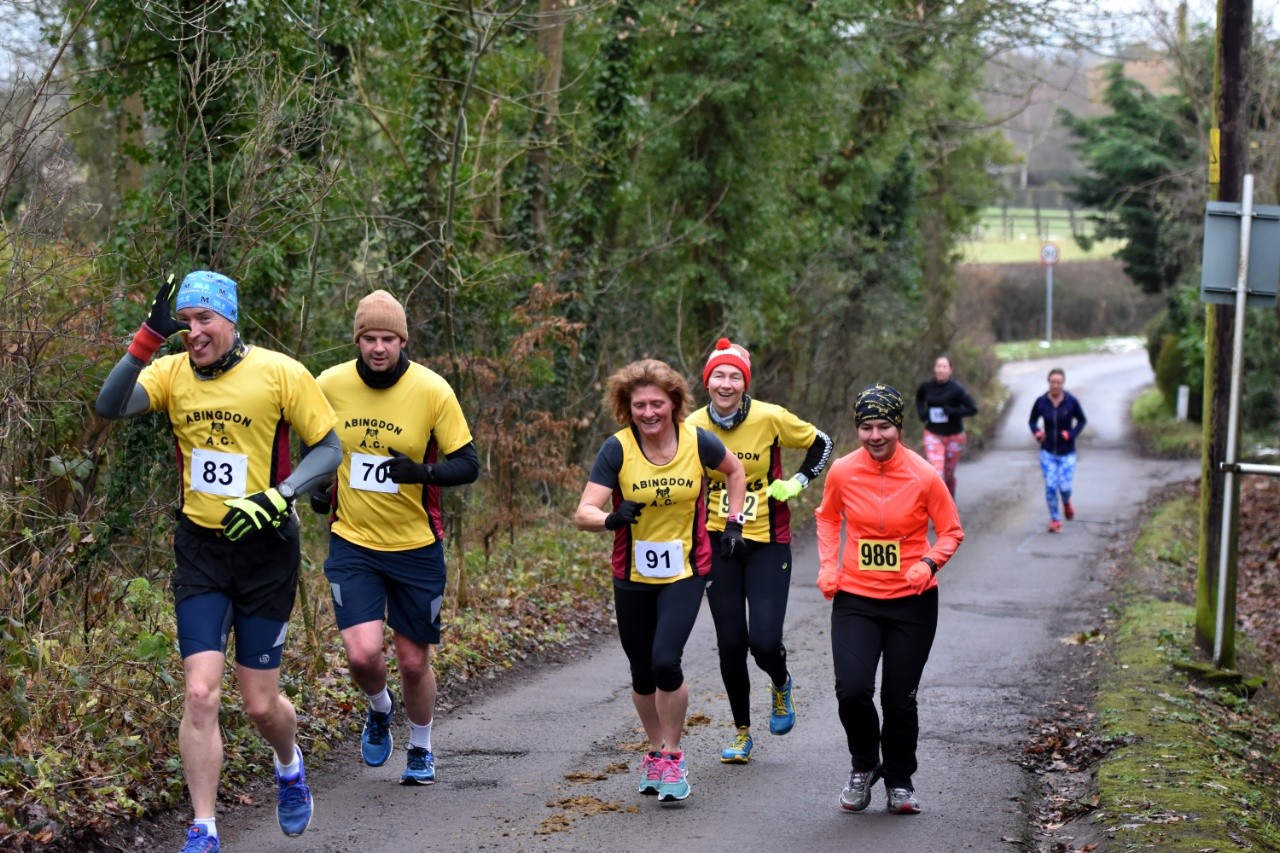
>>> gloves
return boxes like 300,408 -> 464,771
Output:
721,521 -> 746,565
146,274 -> 192,338
309,481 -> 333,515
816,573 -> 839,600
905,561 -> 932,586
220,488 -> 293,542
605,501 -> 646,530
766,479 -> 803,502
1061,430 -> 1069,440
378,447 -> 428,483
1034,431 -> 1046,442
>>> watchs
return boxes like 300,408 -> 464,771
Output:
793,472 -> 810,489
727,513 -> 746,524
919,557 -> 938,575
274,482 -> 297,514
423,464 -> 435,481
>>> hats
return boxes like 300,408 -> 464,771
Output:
703,338 -> 752,393
176,271 -> 238,324
853,383 -> 905,430
353,289 -> 408,344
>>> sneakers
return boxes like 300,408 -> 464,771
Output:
638,750 -> 663,795
273,743 -> 314,837
657,751 -> 690,801
769,671 -> 795,735
839,761 -> 882,811
399,742 -> 436,785
360,689 -> 395,767
721,726 -> 753,762
177,824 -> 220,853
886,787 -> 921,814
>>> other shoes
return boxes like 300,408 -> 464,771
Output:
1064,504 -> 1075,521
1048,520 -> 1063,533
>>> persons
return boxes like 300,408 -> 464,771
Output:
301,290 -> 480,782
96,269 -> 342,853
1029,368 -> 1087,532
685,338 -> 979,817
572,359 -> 747,803
